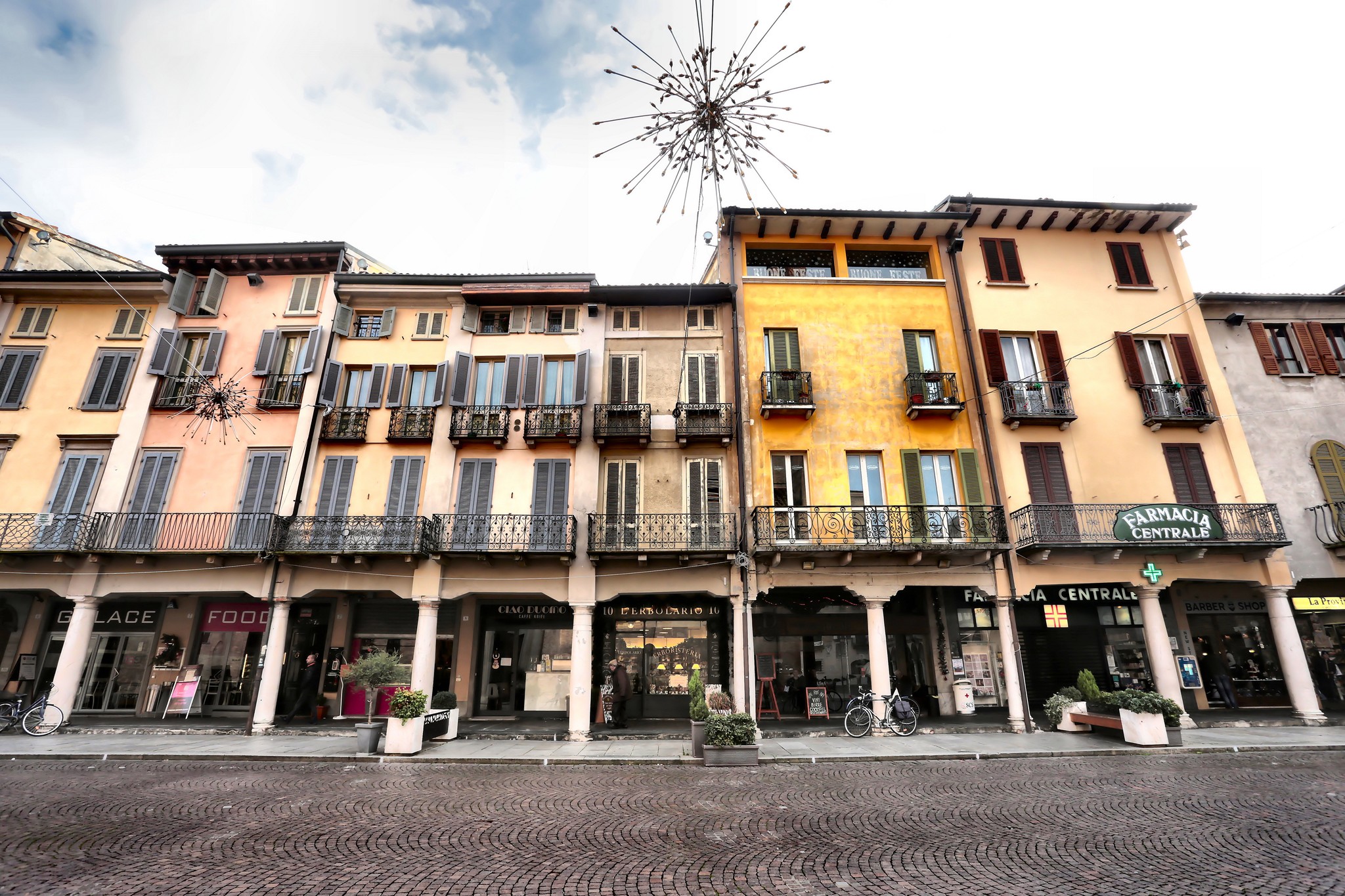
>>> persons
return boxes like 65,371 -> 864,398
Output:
1226,652 -> 1239,679
786,670 -> 807,715
1207,654 -> 1240,710
861,662 -> 871,690
607,659 -> 633,730
1312,650 -> 1341,704
279,654 -> 320,725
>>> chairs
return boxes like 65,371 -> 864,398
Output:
203,678 -> 244,705
74,681 -> 132,709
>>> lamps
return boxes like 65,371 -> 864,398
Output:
588,305 -> 599,318
703,231 -> 717,248
27,230 -> 51,252
357,258 -> 369,270
1225,311 -> 1246,326
246,273 -> 264,287
1176,228 -> 1190,251
166,597 -> 178,609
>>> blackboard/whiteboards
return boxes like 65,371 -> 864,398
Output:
806,687 -> 829,716
755,653 -> 776,680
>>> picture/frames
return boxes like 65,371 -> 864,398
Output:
153,647 -> 186,671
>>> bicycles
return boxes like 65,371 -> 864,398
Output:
792,679 -> 842,712
846,686 -> 920,738
0,681 -> 63,737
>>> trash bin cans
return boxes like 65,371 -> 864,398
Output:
952,678 -> 975,714
144,684 -> 162,712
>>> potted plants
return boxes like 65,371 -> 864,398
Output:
687,667 -> 759,766
310,644 -> 460,756
779,368 -> 809,402
921,369 -> 944,404
1163,379 -> 1195,417
1043,669 -> 1184,748
555,428 -> 567,436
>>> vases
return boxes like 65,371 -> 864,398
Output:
1020,409 -> 1028,414
911,393 -> 924,404
1070,712 -> 1122,730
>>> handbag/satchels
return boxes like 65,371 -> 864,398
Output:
891,702 -> 913,719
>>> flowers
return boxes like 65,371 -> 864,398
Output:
1013,386 -> 1036,410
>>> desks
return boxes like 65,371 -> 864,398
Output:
212,680 -> 239,706
